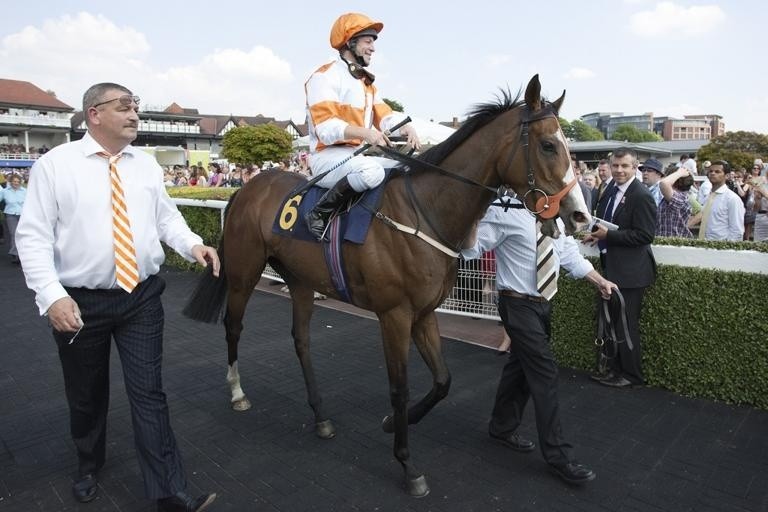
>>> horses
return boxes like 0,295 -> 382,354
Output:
177,73 -> 593,500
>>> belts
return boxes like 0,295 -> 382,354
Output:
497,289 -> 549,305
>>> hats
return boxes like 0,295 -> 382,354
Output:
637,158 -> 665,179
329,13 -> 384,50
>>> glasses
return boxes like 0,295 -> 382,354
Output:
93,94 -> 141,108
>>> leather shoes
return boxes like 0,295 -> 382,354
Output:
71,471 -> 98,502
155,488 -> 217,512
589,369 -> 618,383
598,373 -> 645,390
543,459 -> 597,484
486,418 -> 536,452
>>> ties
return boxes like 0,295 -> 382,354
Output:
96,150 -> 140,294
535,217 -> 559,302
597,185 -> 621,251
698,191 -> 717,241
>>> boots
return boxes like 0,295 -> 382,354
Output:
301,174 -> 363,243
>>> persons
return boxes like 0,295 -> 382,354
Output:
2,142 -> 49,266
572,144 -> 767,246
582,147 -> 655,387
15,83 -> 222,512
164,151 -> 309,187
460,191 -> 618,486
303,13 -> 422,243
